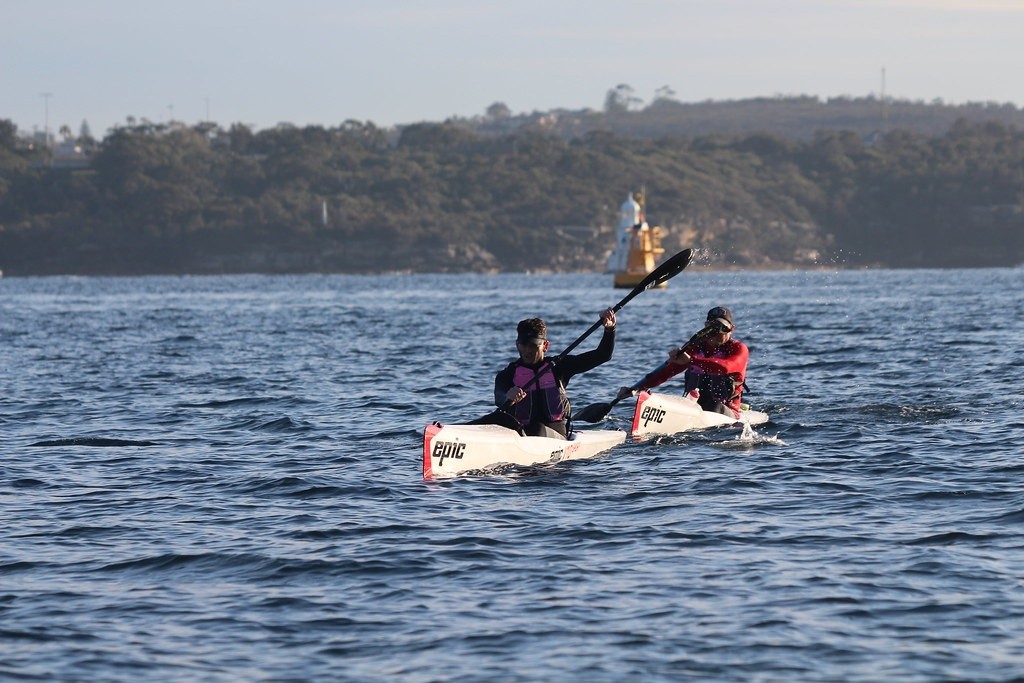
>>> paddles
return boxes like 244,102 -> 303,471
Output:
572,323 -> 723,422
471,247 -> 694,424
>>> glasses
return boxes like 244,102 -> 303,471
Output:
713,326 -> 730,332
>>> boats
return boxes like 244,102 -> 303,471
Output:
612,219 -> 670,289
421,420 -> 627,485
631,388 -> 771,444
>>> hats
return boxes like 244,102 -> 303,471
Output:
517,317 -> 546,345
708,307 -> 733,326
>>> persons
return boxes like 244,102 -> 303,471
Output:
494,307 -> 616,441
617,307 -> 749,419
